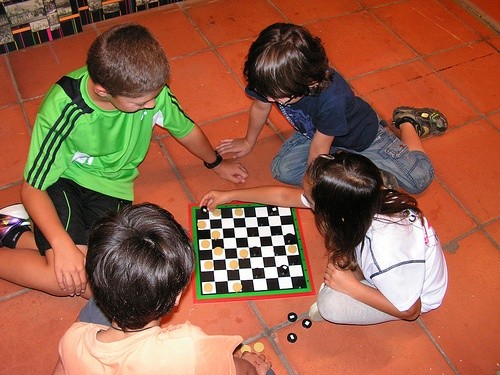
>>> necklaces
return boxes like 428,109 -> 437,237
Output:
110,324 -> 159,333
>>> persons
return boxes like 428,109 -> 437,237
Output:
51,202 -> 276,375
198,150 -> 448,325
216,23 -> 449,194
0,22 -> 249,301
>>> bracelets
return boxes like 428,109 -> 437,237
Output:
204,150 -> 223,169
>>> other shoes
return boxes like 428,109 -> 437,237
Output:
0,204 -> 31,247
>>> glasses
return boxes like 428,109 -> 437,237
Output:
248,80 -> 295,107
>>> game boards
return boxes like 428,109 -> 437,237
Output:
188,200 -> 316,303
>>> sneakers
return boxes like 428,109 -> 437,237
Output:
393,105 -> 448,136
